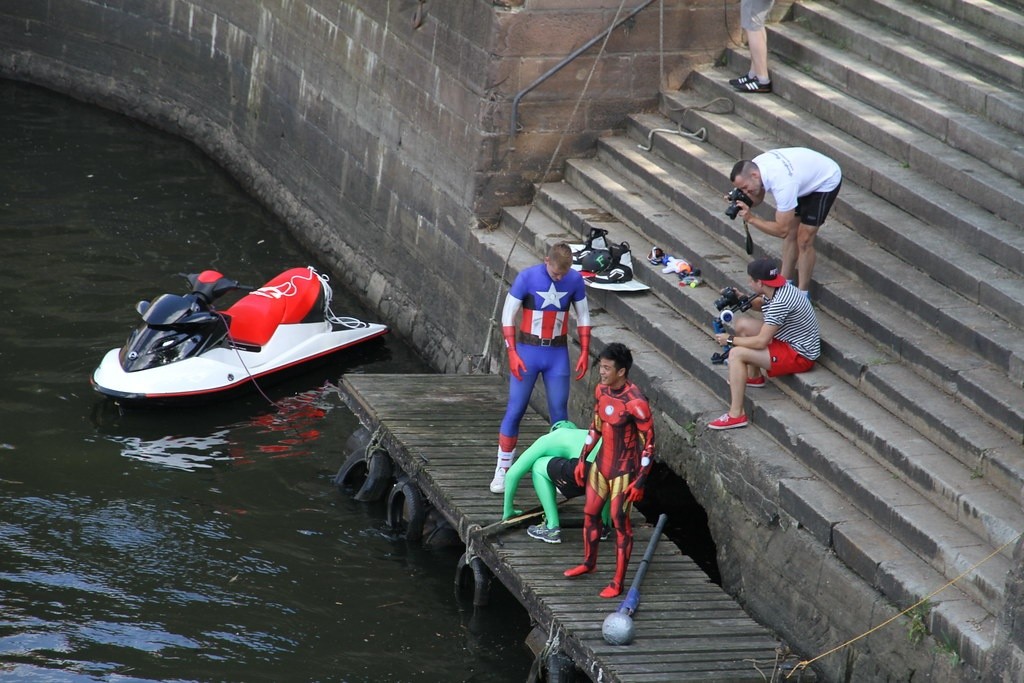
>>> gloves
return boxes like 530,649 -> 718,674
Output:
574,429 -> 601,486
575,326 -> 591,382
624,451 -> 655,504
502,325 -> 527,382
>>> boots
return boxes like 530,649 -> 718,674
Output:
571,228 -> 608,264
595,242 -> 634,284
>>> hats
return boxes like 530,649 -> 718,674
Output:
747,260 -> 786,288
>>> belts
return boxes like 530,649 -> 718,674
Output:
518,331 -> 568,347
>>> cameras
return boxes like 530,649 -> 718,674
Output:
713,286 -> 739,312
725,187 -> 753,220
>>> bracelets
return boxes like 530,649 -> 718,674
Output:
727,336 -> 734,346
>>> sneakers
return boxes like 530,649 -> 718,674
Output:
707,413 -> 749,430
734,77 -> 772,93
489,467 -> 506,494
729,73 -> 751,86
726,375 -> 766,387
600,527 -> 611,540
527,522 -> 562,544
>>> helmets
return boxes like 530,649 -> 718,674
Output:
582,249 -> 612,272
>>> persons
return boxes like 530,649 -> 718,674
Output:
707,259 -> 820,429
490,244 -> 592,494
728,0 -> 775,92
502,420 -> 611,543
725,149 -> 842,301
563,343 -> 655,598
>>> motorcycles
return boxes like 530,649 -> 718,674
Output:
89,267 -> 392,411
